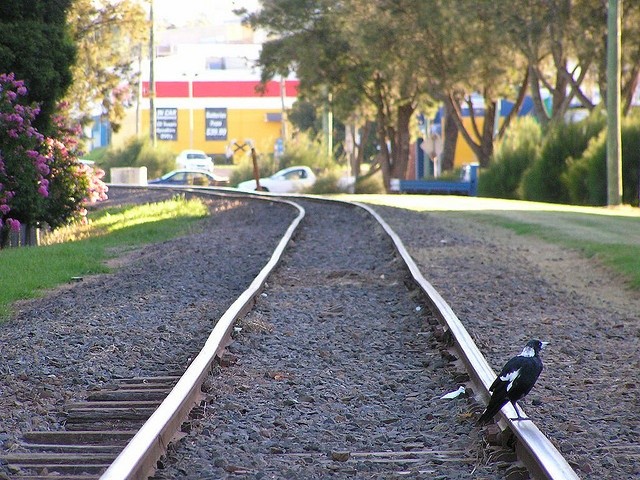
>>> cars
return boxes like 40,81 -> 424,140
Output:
176,150 -> 215,172
238,166 -> 317,193
147,169 -> 229,188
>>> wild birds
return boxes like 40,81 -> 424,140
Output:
474,337 -> 553,429
